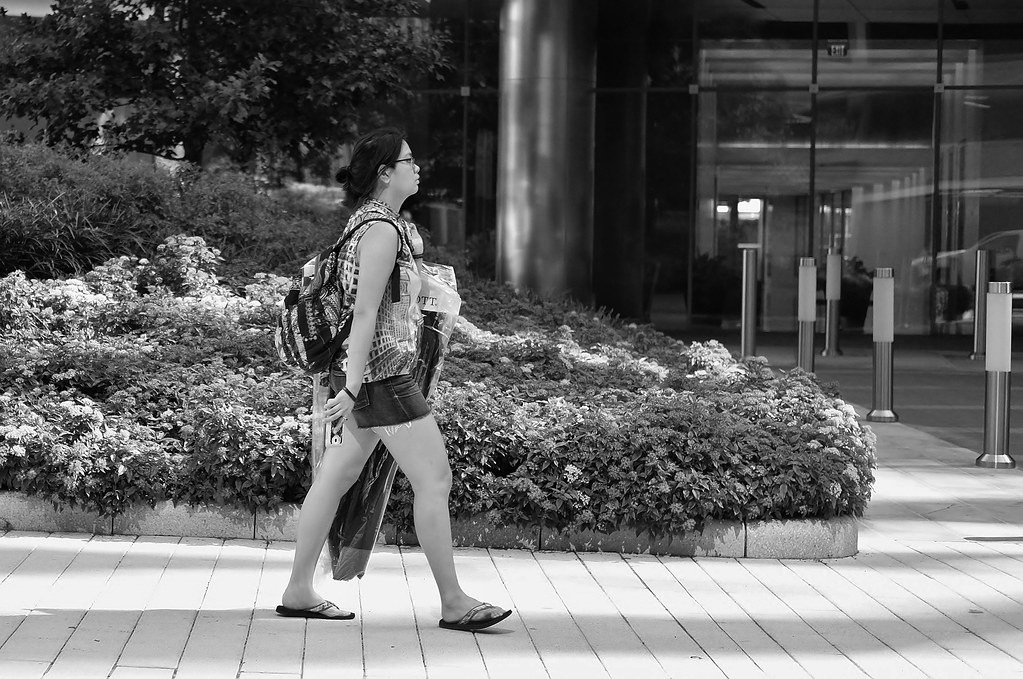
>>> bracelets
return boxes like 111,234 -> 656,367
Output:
342,386 -> 358,404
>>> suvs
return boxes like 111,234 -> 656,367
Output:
912,229 -> 1023,296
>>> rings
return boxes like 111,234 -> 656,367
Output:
342,417 -> 349,421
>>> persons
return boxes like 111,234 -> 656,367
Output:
275,124 -> 512,631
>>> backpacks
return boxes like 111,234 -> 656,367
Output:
275,216 -> 415,378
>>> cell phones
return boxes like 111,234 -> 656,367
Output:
325,398 -> 345,447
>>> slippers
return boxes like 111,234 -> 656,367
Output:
275,600 -> 355,619
438,602 -> 512,630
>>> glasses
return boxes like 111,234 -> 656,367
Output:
394,158 -> 416,168
